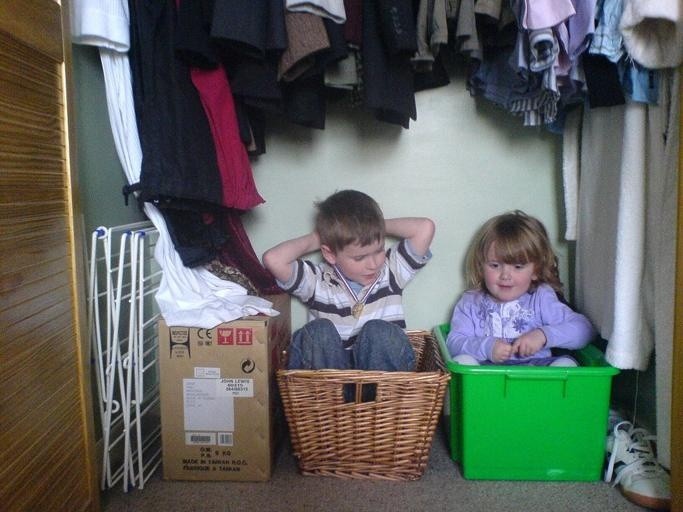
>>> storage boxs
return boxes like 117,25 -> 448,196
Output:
435,324 -> 618,483
160,291 -> 292,482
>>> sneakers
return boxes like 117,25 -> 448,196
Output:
604,421 -> 673,510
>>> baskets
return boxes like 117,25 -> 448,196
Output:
277,330 -> 451,482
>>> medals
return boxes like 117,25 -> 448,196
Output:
350,301 -> 365,320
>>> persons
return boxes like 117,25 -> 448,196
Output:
445,207 -> 596,369
261,186 -> 436,403
